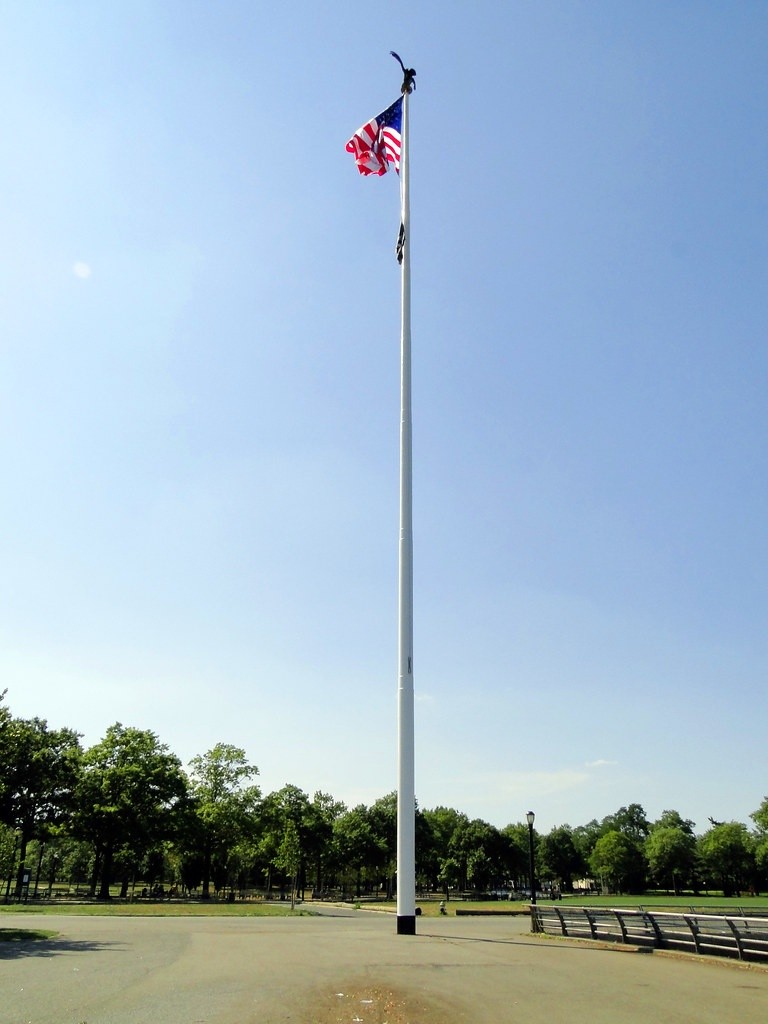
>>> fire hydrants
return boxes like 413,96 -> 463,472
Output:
440,900 -> 446,915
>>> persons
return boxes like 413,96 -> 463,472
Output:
319,885 -> 334,902
141,883 -> 175,904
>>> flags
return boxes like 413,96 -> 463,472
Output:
345,94 -> 402,178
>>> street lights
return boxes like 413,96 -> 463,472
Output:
525,810 -> 542,933
